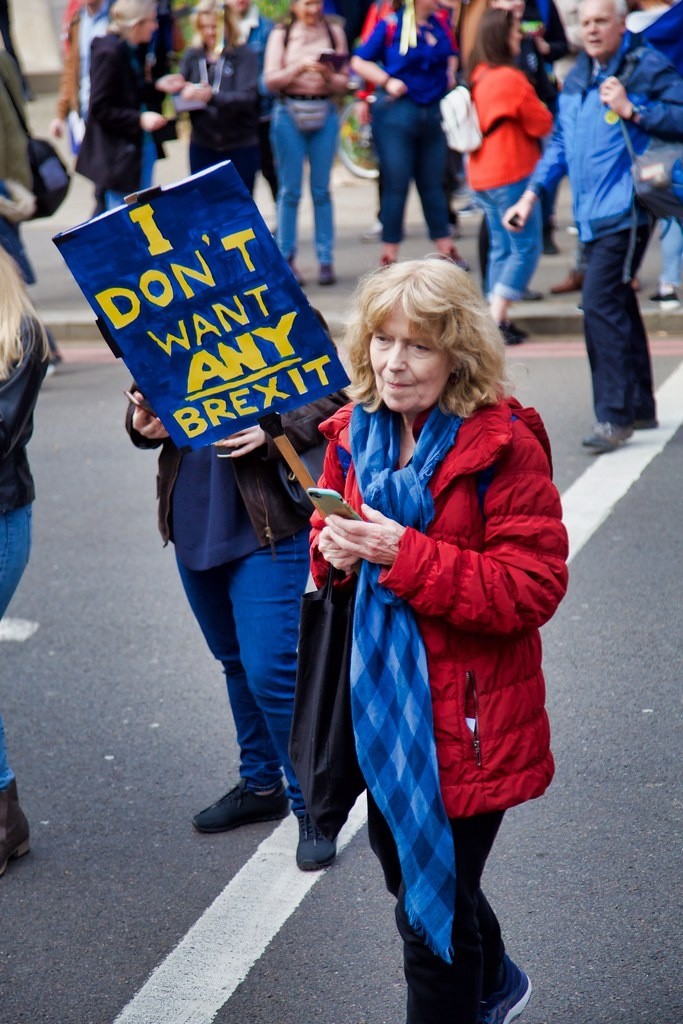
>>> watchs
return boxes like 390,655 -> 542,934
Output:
628,106 -> 639,122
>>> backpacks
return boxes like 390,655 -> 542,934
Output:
439,79 -> 482,152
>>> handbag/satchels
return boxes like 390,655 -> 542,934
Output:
28,139 -> 69,219
289,589 -> 367,840
630,141 -> 683,216
287,98 -> 330,129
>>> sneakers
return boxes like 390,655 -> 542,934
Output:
298,814 -> 336,870
193,778 -> 291,833
479,955 -> 532,1024
582,423 -> 634,448
630,419 -> 657,429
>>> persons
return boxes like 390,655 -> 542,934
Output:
501,0 -> 683,451
125,304 -> 353,871
0,242 -> 50,878
308,252 -> 570,1024
0,0 -> 683,375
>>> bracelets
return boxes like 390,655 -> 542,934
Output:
384,77 -> 391,88
296,62 -> 303,75
520,194 -> 534,205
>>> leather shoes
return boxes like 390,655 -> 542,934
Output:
552,269 -> 585,294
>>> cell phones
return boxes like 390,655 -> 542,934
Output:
306,486 -> 365,520
123,388 -> 158,419
317,48 -> 347,73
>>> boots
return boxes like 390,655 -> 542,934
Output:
1,779 -> 31,874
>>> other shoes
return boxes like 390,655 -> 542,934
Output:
317,266 -> 335,285
542,231 -> 560,255
523,286 -> 543,301
650,293 -> 678,304
500,323 -> 528,344
444,248 -> 471,271
